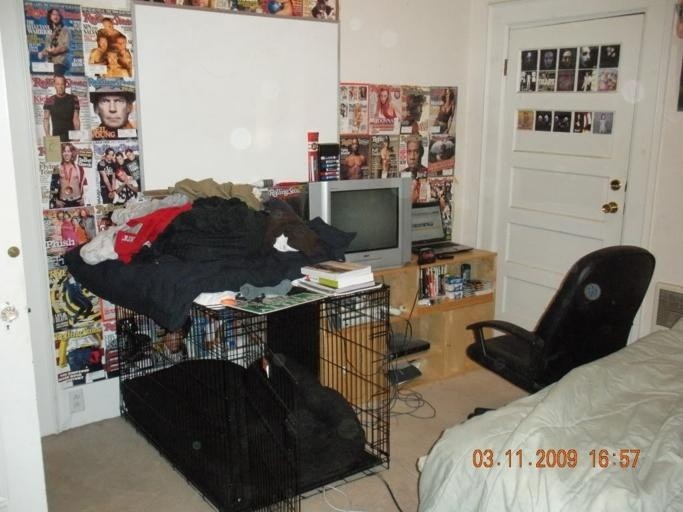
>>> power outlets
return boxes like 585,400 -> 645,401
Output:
69,387 -> 86,414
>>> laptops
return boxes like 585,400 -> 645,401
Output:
412,202 -> 473,256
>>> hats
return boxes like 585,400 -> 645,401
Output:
90,77 -> 135,100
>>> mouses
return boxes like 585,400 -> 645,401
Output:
437,254 -> 454,260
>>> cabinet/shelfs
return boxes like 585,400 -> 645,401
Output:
319,251 -> 497,408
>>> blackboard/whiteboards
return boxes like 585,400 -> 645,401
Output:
129,1 -> 341,197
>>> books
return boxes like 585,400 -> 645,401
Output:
291,259 -> 381,297
419,267 -> 448,298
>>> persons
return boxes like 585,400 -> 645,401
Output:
86,78 -> 136,142
97,148 -> 140,203
377,135 -> 397,178
373,86 -> 396,120
400,135 -> 428,179
559,49 -> 574,70
203,310 -> 224,354
91,17 -> 133,79
341,139 -> 366,180
41,76 -> 81,141
558,72 -> 574,91
434,88 -> 456,135
540,51 -> 555,70
578,47 -> 597,69
400,88 -> 429,135
39,9 -> 71,76
46,143 -> 89,208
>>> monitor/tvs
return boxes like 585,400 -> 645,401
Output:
288,178 -> 413,272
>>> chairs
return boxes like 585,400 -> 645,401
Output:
467,245 -> 655,396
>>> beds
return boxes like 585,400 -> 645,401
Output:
422,283 -> 682,508
